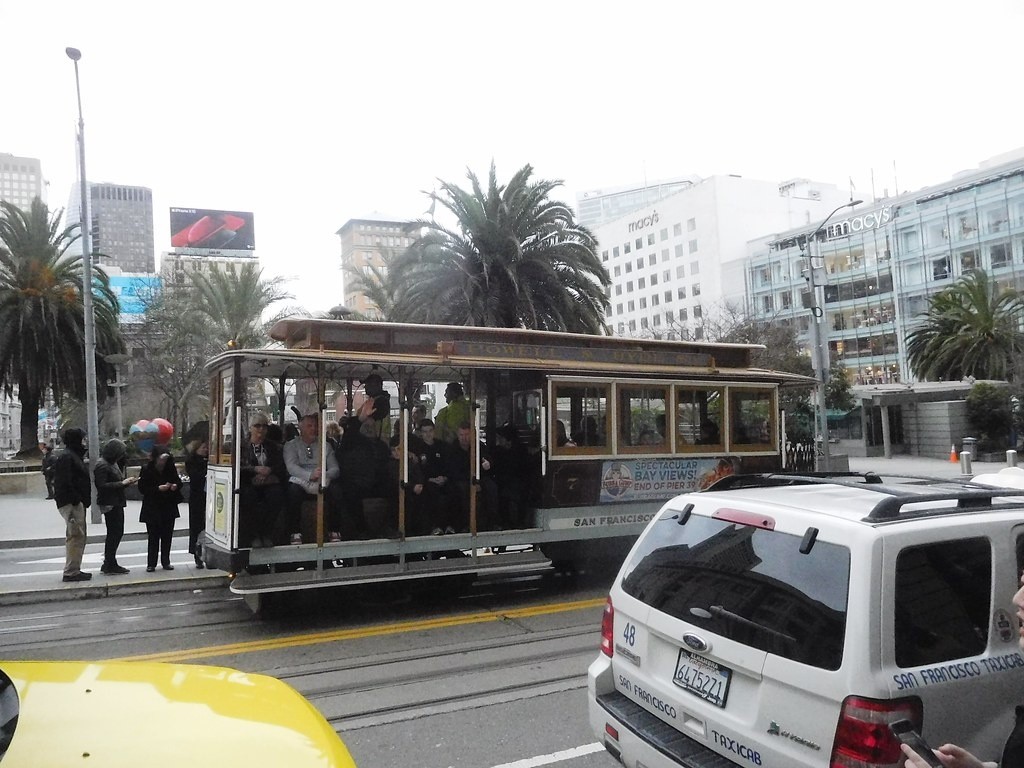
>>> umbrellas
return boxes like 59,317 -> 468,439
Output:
181,420 -> 209,446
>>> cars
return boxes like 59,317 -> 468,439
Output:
0,654 -> 360,768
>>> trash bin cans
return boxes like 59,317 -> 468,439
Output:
962,437 -> 978,462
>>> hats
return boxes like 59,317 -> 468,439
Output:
184,438 -> 202,455
42,443 -> 53,450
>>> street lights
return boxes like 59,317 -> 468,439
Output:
66,46 -> 106,524
804,199 -> 864,471
104,354 -> 131,441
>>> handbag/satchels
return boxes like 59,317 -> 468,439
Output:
251,472 -> 280,486
359,416 -> 376,439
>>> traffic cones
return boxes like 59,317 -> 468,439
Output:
948,444 -> 959,463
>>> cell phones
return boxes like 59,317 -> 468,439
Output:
171,215 -> 245,248
133,477 -> 141,482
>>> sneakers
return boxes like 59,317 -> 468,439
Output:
429,528 -> 455,535
289,532 -> 303,544
328,530 -> 342,541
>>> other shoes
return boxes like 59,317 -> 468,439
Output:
146,565 -> 155,572
45,495 -> 53,499
246,536 -> 274,549
63,570 -> 92,582
100,562 -> 130,575
195,562 -> 204,568
163,564 -> 174,570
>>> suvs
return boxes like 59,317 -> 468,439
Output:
586,473 -> 1023,768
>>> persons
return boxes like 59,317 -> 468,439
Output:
138,448 -> 183,572
185,440 -> 210,569
239,374 -> 540,549
558,420 -> 575,446
41,447 -> 56,499
639,414 -> 687,444
94,438 -> 134,573
695,421 -> 720,445
54,428 -> 92,582
901,570 -> 1024,768
576,416 -> 606,446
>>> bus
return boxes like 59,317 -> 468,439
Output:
190,318 -> 823,615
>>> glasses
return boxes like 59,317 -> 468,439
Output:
249,424 -> 267,429
304,446 -> 313,458
